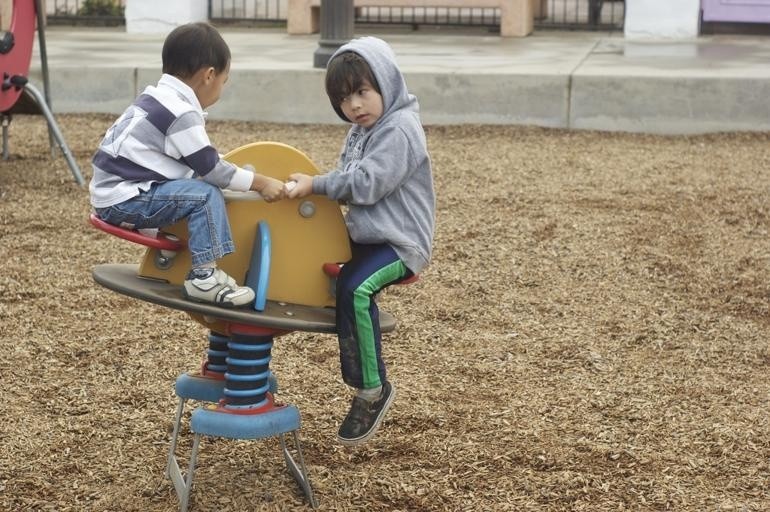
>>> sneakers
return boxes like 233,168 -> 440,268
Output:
180,268 -> 256,308
337,380 -> 395,448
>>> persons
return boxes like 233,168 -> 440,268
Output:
288,36 -> 436,445
89,22 -> 287,308
